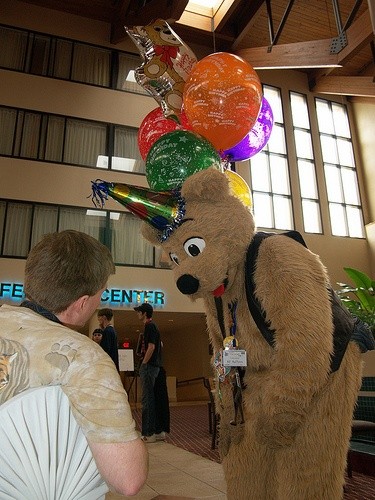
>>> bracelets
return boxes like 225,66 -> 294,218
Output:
140,362 -> 147,369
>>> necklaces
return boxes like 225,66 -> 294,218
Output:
143,318 -> 153,325
106,324 -> 113,327
214,296 -> 250,426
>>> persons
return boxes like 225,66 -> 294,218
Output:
134,303 -> 170,442
0,229 -> 150,500
92,307 -> 120,373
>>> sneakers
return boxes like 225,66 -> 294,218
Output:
156,432 -> 166,439
140,433 -> 156,442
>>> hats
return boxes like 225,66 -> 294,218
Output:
134,303 -> 153,312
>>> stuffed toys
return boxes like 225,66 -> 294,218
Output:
88,166 -> 364,500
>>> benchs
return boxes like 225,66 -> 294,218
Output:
347,376 -> 375,480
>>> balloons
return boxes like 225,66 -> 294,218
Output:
124,18 -> 274,212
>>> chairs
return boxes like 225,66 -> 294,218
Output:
205,380 -> 219,450
